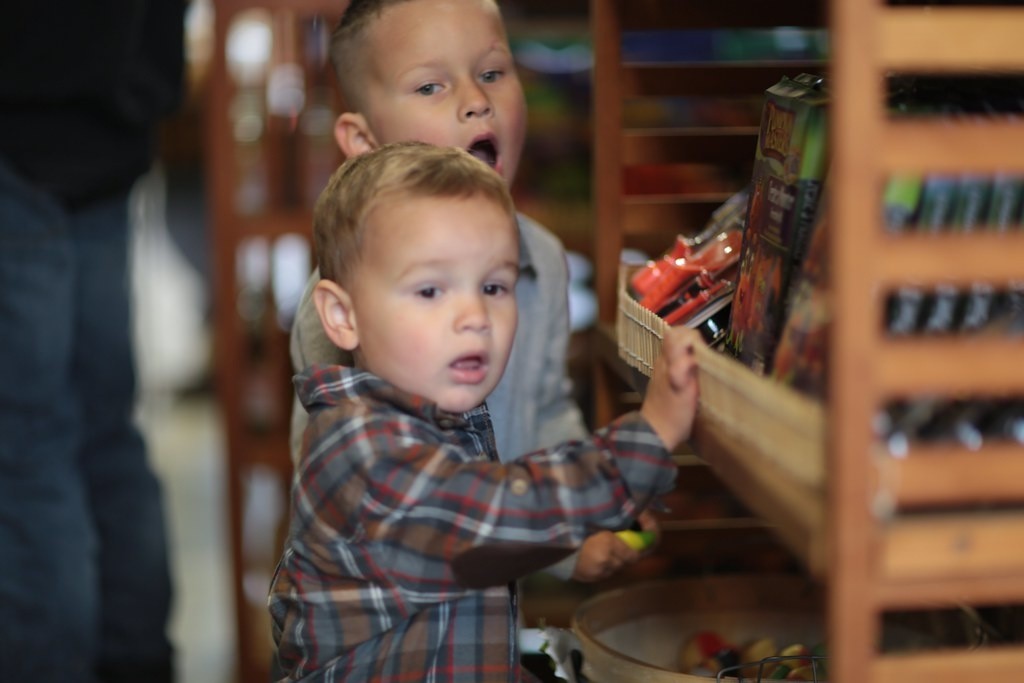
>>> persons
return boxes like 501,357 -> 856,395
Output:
267,139 -> 700,682
0,0 -> 189,682
287,1 -> 663,588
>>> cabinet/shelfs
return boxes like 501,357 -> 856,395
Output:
201,0 -> 1023,679
